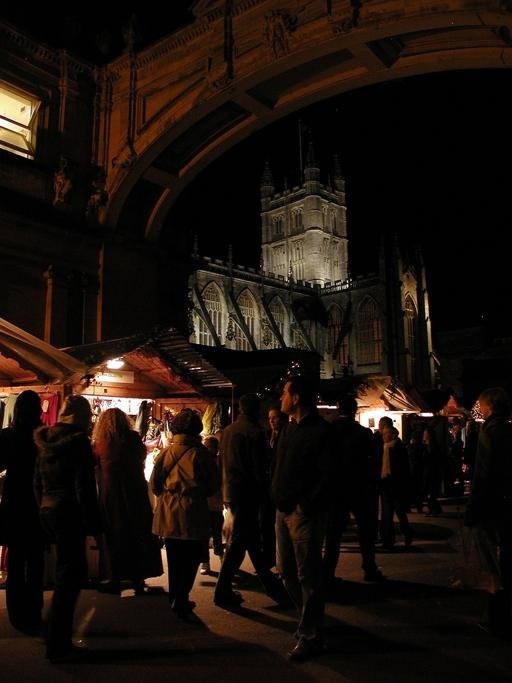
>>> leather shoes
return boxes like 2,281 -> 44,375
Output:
287,635 -> 319,659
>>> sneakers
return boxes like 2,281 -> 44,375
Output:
200,563 -> 210,574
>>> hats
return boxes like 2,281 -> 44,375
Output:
170,411 -> 203,434
57,395 -> 92,425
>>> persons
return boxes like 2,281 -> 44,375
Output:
36,395 -> 100,661
323,392 -> 385,581
274,376 -> 342,663
150,392 -> 291,612
372,411 -> 481,552
471,387 -> 512,598
0,388 -> 165,634
